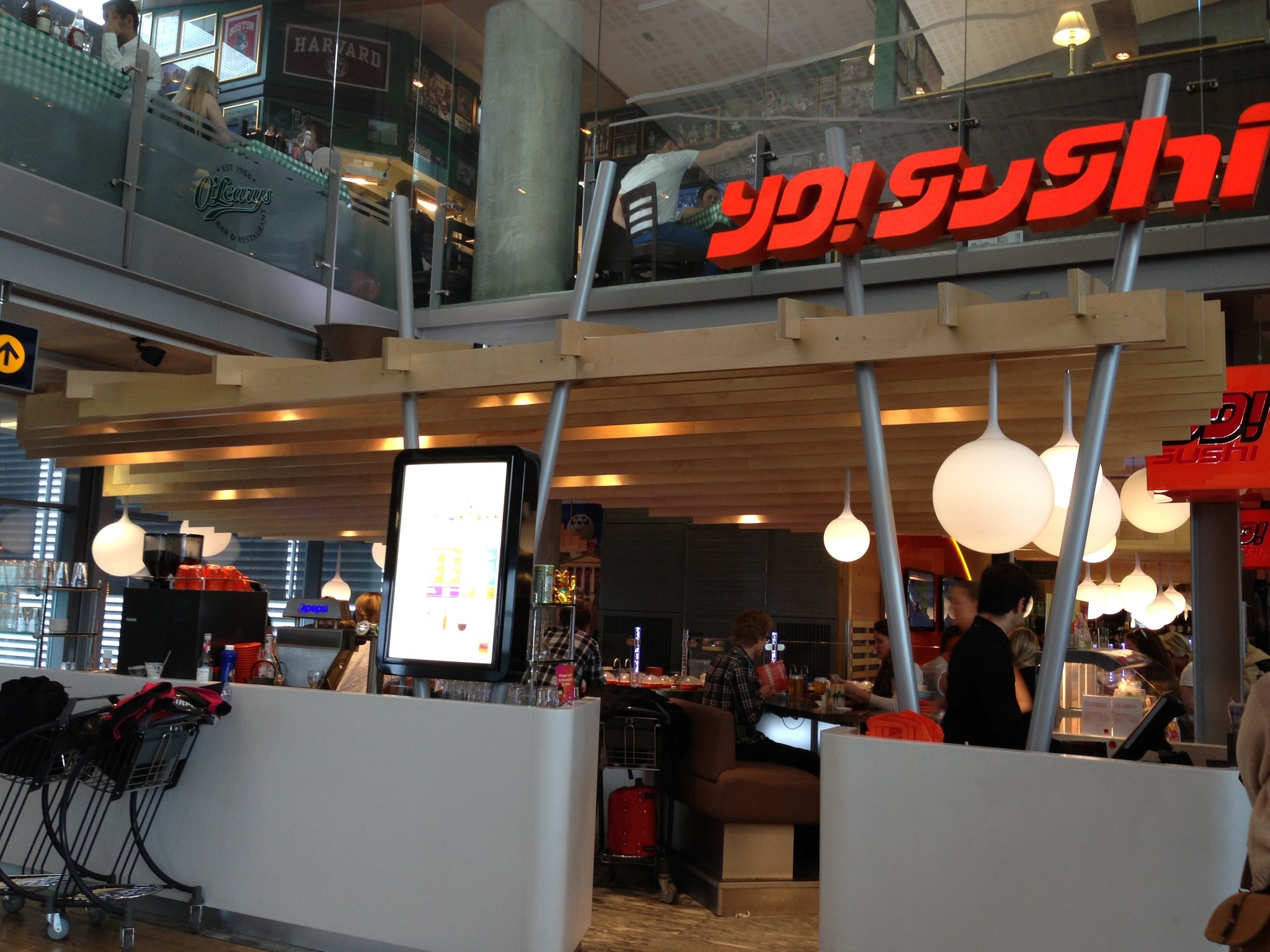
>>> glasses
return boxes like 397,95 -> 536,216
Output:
215,85 -> 221,92
762,635 -> 770,643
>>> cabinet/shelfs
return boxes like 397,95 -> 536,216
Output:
0,559 -> 101,673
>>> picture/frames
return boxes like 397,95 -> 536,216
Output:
579,2 -> 944,187
137,1 -> 480,198
904,568 -> 937,632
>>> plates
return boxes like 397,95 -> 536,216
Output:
606,679 -> 704,690
232,642 -> 260,685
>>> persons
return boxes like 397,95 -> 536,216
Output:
169,66 -> 231,147
828,557 -> 1270,952
330,591 -> 388,693
698,607 -> 791,763
96,0 -> 163,110
519,603 -> 609,702
609,125 -> 768,271
680,182 -> 722,217
294,119 -> 338,181
369,179 -> 439,249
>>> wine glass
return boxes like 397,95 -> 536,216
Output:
534,617 -> 550,662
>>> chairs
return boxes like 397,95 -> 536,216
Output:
619,181 -> 698,286
409,217 -> 475,308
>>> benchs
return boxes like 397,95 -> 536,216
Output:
667,695 -> 821,881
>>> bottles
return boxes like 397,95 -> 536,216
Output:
258,633 -> 283,685
196,633 -> 214,682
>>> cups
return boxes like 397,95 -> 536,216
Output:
788,674 -> 803,706
815,677 -> 828,695
130,666 -> 146,679
0,559 -> 88,588
172,565 -> 250,591
573,687 -> 579,702
102,650 -> 112,668
60,662 -> 76,671
0,592 -> 67,635
440,679 -> 560,709
307,670 -> 325,689
146,662 -> 164,678
831,683 -> 846,714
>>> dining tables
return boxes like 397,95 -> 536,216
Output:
754,685 -> 894,760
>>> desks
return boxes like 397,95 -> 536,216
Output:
679,197 -> 740,238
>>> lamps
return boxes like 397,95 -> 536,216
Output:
91,496 -> 151,582
824,468 -> 871,563
370,541 -> 386,571
177,519 -> 234,559
1051,10 -> 1094,77
927,356 -> 1190,630
319,543 -> 351,603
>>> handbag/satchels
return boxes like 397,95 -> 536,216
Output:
1204,891 -> 1270,952
